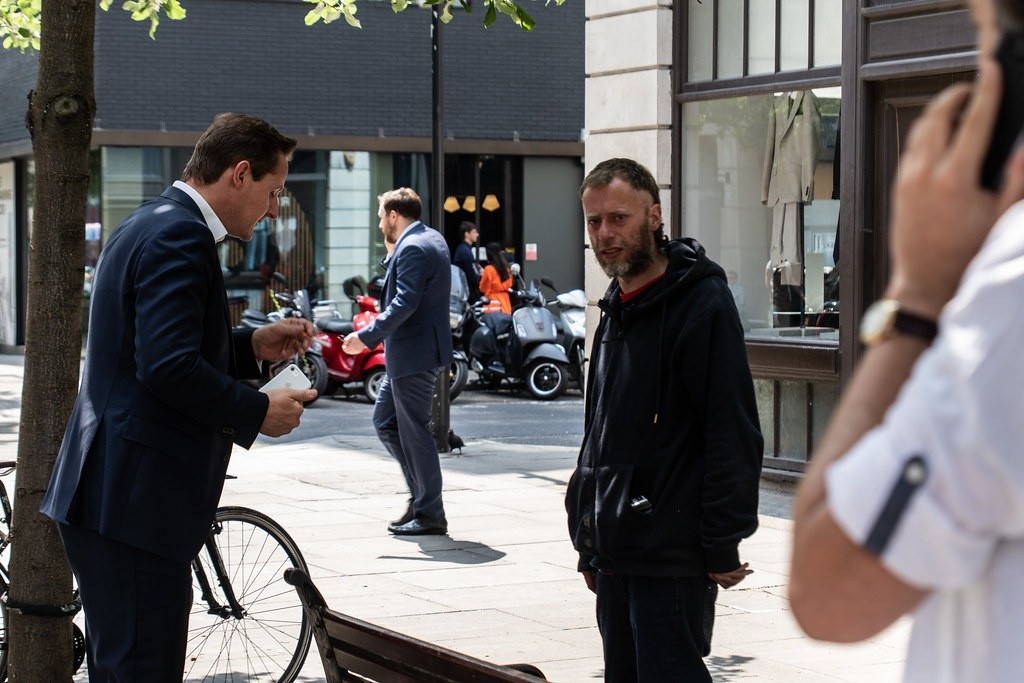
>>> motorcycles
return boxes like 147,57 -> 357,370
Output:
305,266 -> 392,404
365,263 -> 490,401
232,288 -> 327,408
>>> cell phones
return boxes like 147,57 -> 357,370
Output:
947,31 -> 1024,194
259,363 -> 311,394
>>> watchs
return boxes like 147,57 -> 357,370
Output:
860,298 -> 937,343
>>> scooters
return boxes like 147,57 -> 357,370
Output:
468,263 -> 572,401
540,277 -> 588,397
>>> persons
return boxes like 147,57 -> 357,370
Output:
342,188 -> 455,534
453,221 -> 513,316
788,0 -> 1024,683
39,113 -> 317,683
565,158 -> 764,683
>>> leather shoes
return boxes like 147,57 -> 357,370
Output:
388,517 -> 448,536
392,498 -> 414,525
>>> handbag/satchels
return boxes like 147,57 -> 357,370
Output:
483,313 -> 513,334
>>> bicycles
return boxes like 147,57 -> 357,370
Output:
0,439 -> 314,683
